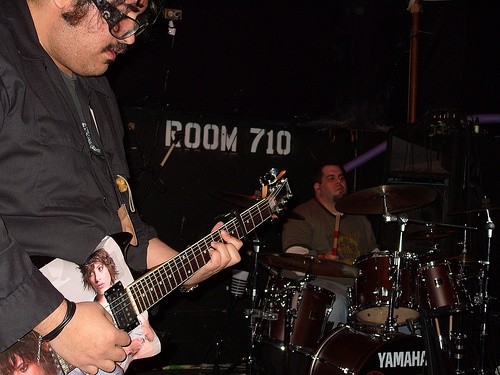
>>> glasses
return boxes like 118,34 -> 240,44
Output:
93,0 -> 149,40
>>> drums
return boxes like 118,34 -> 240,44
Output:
415,258 -> 476,320
309,322 -> 447,375
250,272 -> 337,358
350,250 -> 427,328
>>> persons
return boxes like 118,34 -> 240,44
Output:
283,163 -> 378,267
0,0 -> 243,375
78,248 -> 162,371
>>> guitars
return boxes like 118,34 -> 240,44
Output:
0,168 -> 293,375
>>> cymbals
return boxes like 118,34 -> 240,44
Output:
402,225 -> 462,241
447,207 -> 500,217
199,187 -> 307,221
334,182 -> 439,216
256,252 -> 366,279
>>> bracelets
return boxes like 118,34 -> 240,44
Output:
42,298 -> 76,343
